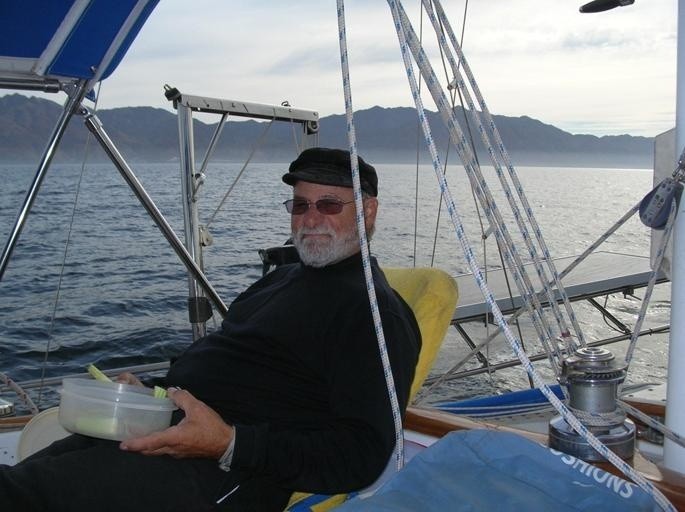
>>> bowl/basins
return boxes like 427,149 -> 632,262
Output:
57,378 -> 181,444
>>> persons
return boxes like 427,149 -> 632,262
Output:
0,148 -> 421,511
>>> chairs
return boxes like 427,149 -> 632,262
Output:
15,269 -> 459,512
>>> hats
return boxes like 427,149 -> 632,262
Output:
282,147 -> 379,200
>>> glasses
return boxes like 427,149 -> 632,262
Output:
283,195 -> 353,217
